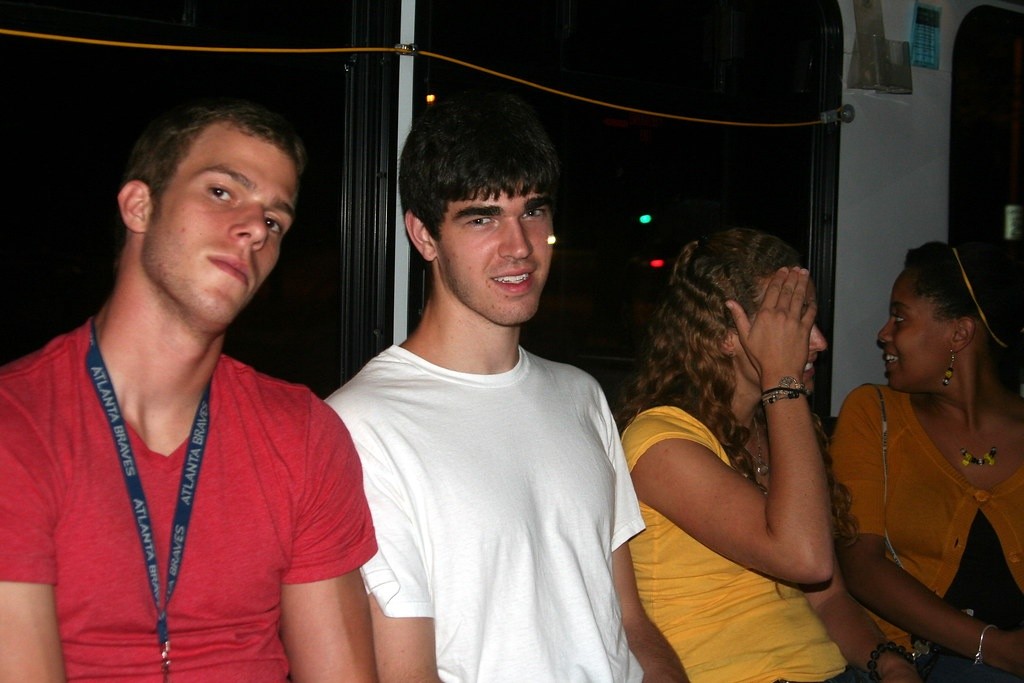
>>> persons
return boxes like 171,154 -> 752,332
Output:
327,101 -> 689,683
0,105 -> 381,683
827,240 -> 1024,683
607,227 -> 923,683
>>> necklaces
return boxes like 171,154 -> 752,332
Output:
959,447 -> 999,466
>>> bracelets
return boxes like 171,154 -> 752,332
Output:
973,623 -> 997,665
762,376 -> 812,405
868,641 -> 919,683
